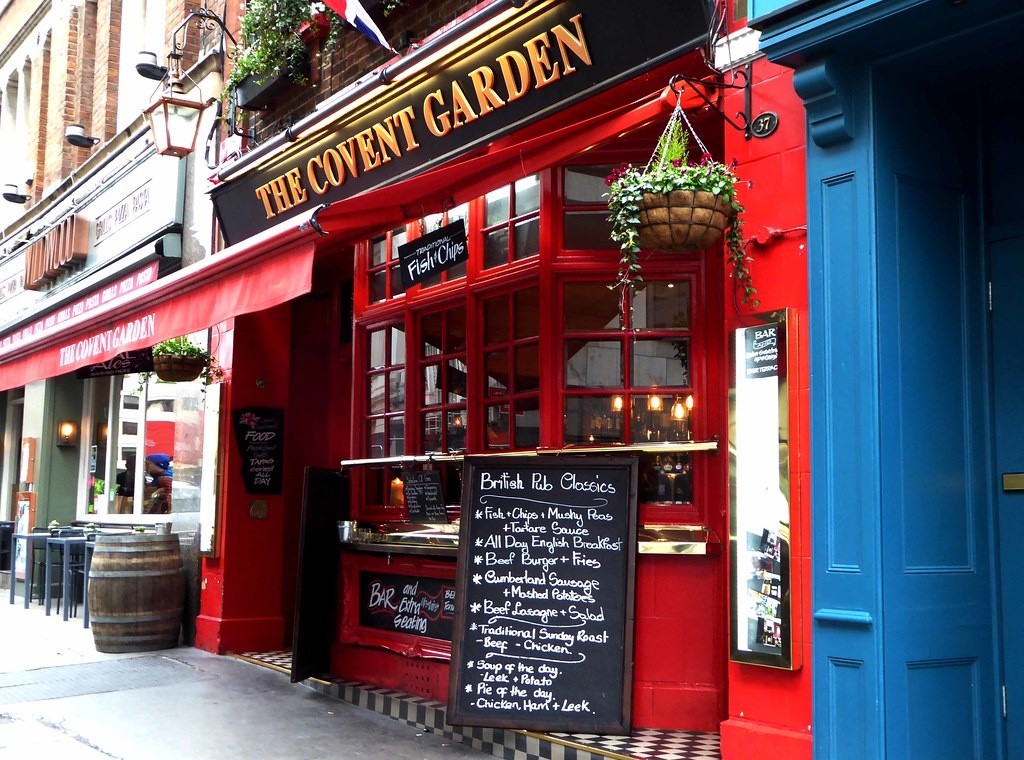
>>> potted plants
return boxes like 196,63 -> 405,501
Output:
134,525 -> 146,532
151,335 -> 222,385
216,0 -> 341,112
47,520 -> 59,534
82,523 -> 97,538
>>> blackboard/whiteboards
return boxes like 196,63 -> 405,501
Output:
402,469 -> 449,524
443,453 -> 642,737
287,462 -> 351,684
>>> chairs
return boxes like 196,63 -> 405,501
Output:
69,532 -> 133,627
57,529 -> 85,619
42,529 -> 67,616
30,527 -> 60,605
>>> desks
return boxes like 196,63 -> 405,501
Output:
45,536 -> 96,621
10,533 -> 52,609
83,542 -> 95,628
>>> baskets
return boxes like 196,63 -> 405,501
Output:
633,189 -> 733,248
153,355 -> 204,382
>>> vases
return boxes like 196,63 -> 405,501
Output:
618,190 -> 732,250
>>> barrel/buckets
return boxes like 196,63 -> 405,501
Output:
88,536 -> 184,652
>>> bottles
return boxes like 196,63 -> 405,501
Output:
590,410 -> 621,433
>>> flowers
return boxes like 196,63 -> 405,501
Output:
602,121 -> 757,313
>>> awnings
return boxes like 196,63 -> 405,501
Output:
1,79 -> 718,394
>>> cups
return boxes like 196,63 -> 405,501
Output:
339,521 -> 354,542
359,529 -> 371,543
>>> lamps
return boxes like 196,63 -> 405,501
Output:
57,420 -> 78,446
145,51 -> 207,158
611,382 -> 695,476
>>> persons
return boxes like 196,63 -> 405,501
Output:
115,455 -> 136,497
145,453 -> 175,514
